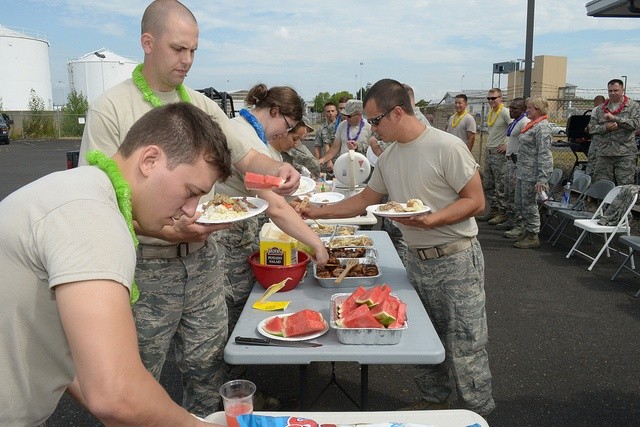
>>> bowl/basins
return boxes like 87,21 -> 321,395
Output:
247,250 -> 311,293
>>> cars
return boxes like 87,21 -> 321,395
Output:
0,113 -> 14,144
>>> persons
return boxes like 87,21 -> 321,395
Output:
76,1 -> 302,418
0,101 -> 232,427
318,99 -> 372,180
281,115 -> 321,181
313,101 -> 338,180
476,87 -> 513,224
445,93 -> 477,151
495,97 -> 530,239
586,95 -> 606,203
588,79 -> 640,209
368,83 -> 432,158
211,82 -> 329,334
321,96 -> 350,172
264,119 -> 306,166
290,78 -> 496,418
511,95 -> 554,249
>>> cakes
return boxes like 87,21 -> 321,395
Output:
407,199 -> 423,210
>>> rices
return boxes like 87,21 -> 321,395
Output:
203,204 -> 239,221
377,204 -> 407,213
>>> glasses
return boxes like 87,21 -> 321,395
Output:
487,96 -> 499,100
282,114 -> 293,132
366,102 -> 404,126
343,113 -> 358,118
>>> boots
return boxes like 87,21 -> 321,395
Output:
395,398 -> 450,410
513,233 -> 541,249
496,222 -> 514,231
475,207 -> 498,222
488,214 -> 505,224
503,226 -> 527,238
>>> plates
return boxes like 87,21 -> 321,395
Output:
365,203 -> 431,217
299,191 -> 345,205
196,196 -> 269,224
257,312 -> 330,342
325,180 -> 334,185
290,175 -> 316,196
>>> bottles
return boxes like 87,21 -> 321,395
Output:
321,185 -> 325,192
561,182 -> 571,208
534,184 -> 549,202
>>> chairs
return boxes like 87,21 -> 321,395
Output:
541,174 -> 591,243
566,185 -> 638,272
539,168 -> 563,224
610,236 -> 640,298
552,179 -> 615,256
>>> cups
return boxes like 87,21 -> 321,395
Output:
219,378 -> 256,427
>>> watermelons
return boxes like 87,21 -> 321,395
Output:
283,320 -> 325,338
336,283 -> 406,330
283,309 -> 322,328
244,172 -> 285,191
263,316 -> 282,336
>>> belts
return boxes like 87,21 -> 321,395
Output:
408,235 -> 478,261
136,234 -> 212,259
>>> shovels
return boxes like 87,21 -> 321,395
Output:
335,259 -> 359,284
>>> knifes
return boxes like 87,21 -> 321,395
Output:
235,336 -> 324,348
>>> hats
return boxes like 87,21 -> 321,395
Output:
341,98 -> 364,116
302,115 -> 314,133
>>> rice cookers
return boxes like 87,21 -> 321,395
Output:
332,149 -> 372,199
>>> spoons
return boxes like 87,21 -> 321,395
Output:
313,218 -> 326,229
